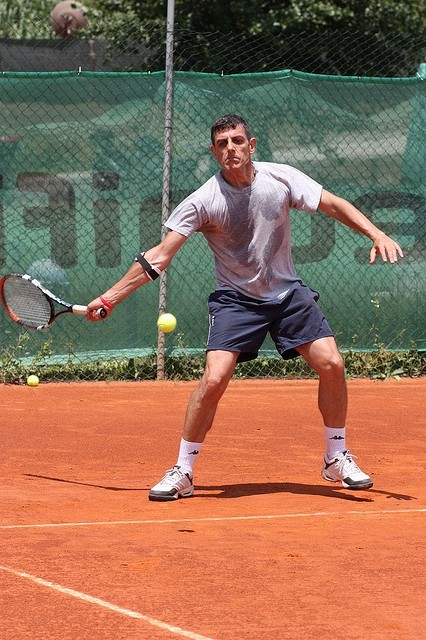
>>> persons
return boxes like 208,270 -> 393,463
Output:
90,113 -> 406,501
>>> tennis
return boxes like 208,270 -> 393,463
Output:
26,374 -> 39,386
157,313 -> 178,333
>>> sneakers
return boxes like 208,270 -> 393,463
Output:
149,466 -> 194,501
322,451 -> 373,490
